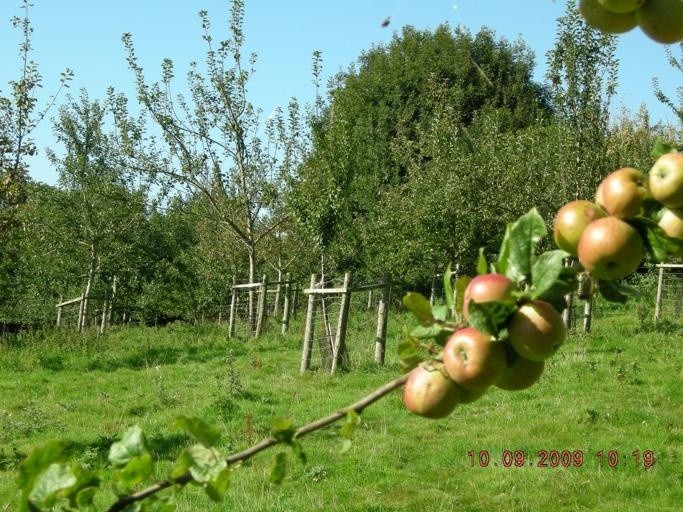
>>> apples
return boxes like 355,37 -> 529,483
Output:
401,273 -> 565,419
552,151 -> 683,281
